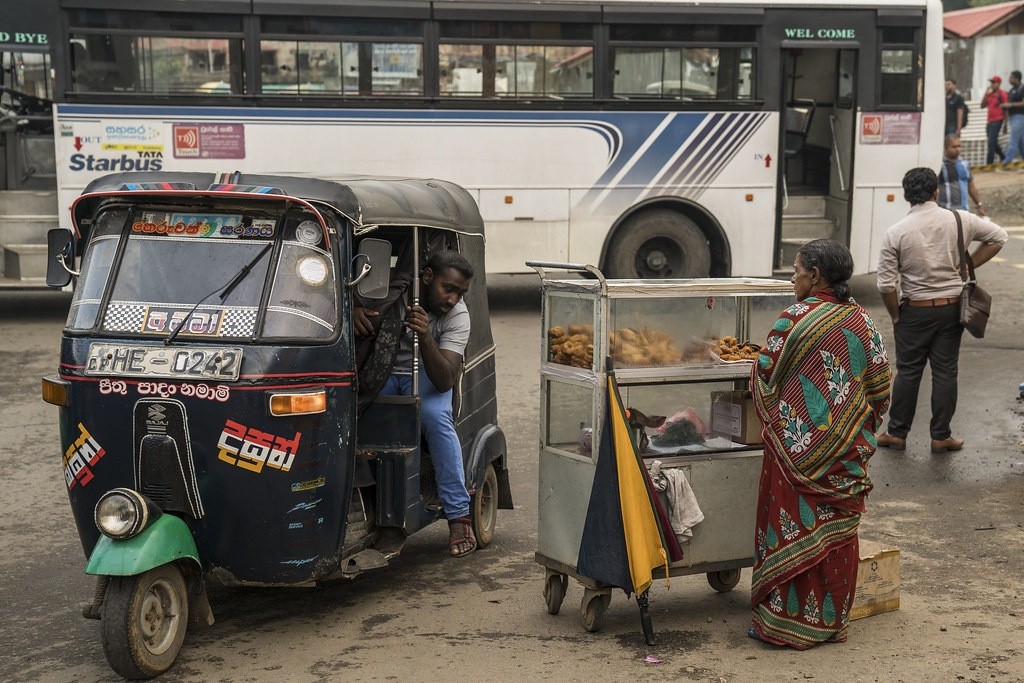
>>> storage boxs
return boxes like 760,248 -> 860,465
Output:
847,538 -> 901,621
710,390 -> 764,445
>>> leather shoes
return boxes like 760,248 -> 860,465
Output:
874,430 -> 906,450
932,436 -> 965,452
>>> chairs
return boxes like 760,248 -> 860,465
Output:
22,42 -> 87,135
784,97 -> 816,187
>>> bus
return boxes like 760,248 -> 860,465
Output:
0,0 -> 944,295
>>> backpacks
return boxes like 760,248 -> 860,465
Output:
950,91 -> 968,127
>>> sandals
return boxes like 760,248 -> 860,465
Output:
447,513 -> 477,557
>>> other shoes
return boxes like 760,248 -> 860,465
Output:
748,626 -> 763,641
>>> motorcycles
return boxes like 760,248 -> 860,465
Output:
41,170 -> 516,683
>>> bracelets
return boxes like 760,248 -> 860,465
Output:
976,202 -> 982,208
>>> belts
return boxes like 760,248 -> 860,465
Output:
901,298 -> 960,307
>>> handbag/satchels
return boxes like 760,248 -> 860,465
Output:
959,281 -> 991,338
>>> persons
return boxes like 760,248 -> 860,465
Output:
945,79 -> 964,138
1000,70 -> 1024,169
980,76 -> 1006,172
750,239 -> 891,650
353,251 -> 478,558
878,167 -> 1008,453
936,133 -> 986,217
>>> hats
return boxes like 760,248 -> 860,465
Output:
989,76 -> 1001,82
